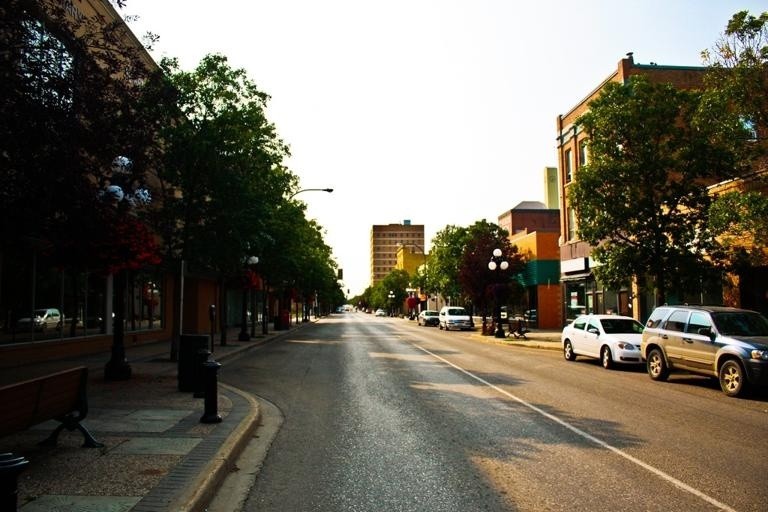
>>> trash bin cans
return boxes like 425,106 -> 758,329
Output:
1,454 -> 29,512
179,334 -> 213,393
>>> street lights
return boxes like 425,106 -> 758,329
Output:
487,247 -> 509,338
93,156 -> 153,381
396,244 -> 428,312
260,187 -> 335,334
388,290 -> 395,317
236,243 -> 259,341
406,282 -> 418,319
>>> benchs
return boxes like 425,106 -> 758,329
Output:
0,365 -> 106,454
507,320 -> 531,341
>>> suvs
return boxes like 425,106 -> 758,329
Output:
640,304 -> 767,397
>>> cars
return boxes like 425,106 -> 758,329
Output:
562,314 -> 646,369
336,302 -> 354,313
361,307 -> 384,317
17,308 -> 126,332
244,308 -> 262,321
524,310 -> 536,322
418,304 -> 470,332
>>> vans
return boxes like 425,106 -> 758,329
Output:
499,305 -> 507,319
